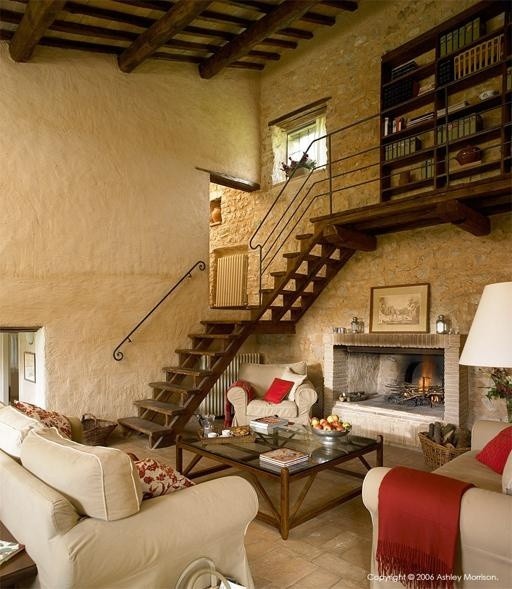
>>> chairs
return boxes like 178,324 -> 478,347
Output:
361,419 -> 512,588
225,362 -> 318,427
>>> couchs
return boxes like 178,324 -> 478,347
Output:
0,401 -> 259,589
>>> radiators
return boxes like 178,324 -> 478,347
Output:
213,254 -> 247,307
199,352 -> 260,416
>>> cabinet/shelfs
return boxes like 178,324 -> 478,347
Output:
380,0 -> 512,202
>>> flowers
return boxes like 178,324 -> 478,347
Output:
282,152 -> 315,178
480,368 -> 512,422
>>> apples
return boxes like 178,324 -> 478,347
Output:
311,415 -> 350,432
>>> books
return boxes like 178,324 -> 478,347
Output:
0,539 -> 25,565
383,18 -> 512,180
259,447 -> 309,468
250,415 -> 289,437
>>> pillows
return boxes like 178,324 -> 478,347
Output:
134,456 -> 195,498
264,367 -> 307,404
476,425 -> 512,475
0,405 -> 42,461
14,399 -> 71,439
20,426 -> 142,522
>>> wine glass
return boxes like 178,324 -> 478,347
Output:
311,442 -> 350,465
311,428 -> 351,443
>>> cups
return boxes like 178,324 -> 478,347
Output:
222,429 -> 232,438
207,432 -> 219,438
332,327 -> 356,334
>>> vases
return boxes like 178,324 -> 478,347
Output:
506,395 -> 512,423
293,167 -> 310,178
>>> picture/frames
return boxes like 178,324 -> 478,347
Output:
370,282 -> 430,334
24,352 -> 36,383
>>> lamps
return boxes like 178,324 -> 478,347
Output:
458,281 -> 512,369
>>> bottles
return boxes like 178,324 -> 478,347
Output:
350,316 -> 359,333
202,412 -> 214,438
435,315 -> 448,335
